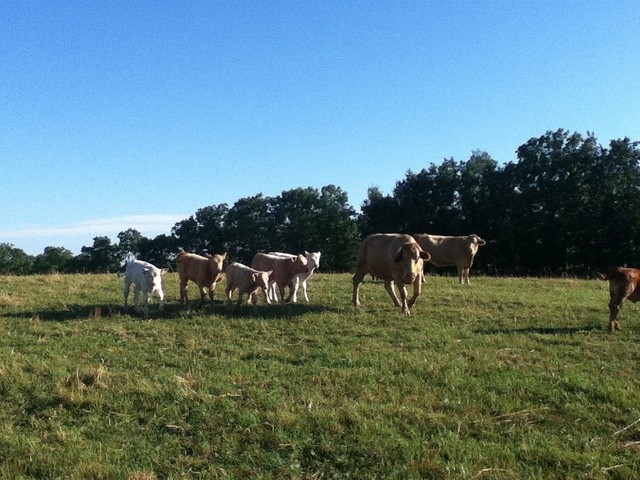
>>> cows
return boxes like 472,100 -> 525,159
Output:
281,306 -> 282,306
267,251 -> 322,303
177,246 -> 227,309
225,261 -> 273,309
352,233 -> 431,316
412,233 -> 486,285
123,259 -> 170,320
252,253 -> 309,306
605,267 -> 640,332
